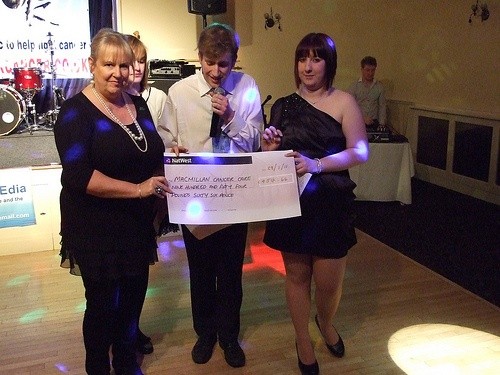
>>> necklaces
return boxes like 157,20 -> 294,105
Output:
89,83 -> 148,152
301,86 -> 327,105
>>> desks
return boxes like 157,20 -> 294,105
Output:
348,127 -> 415,206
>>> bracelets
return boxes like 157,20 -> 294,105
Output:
315,158 -> 322,174
137,183 -> 141,199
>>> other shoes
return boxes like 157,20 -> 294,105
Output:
135,328 -> 153,354
219,336 -> 246,368
315,313 -> 345,358
192,335 -> 217,364
295,338 -> 319,375
113,359 -> 143,375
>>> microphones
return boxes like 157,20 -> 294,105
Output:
260,95 -> 272,126
209,87 -> 225,137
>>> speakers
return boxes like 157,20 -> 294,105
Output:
187,0 -> 226,15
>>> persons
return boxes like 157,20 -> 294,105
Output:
169,24 -> 262,368
55,30 -> 189,375
259,33 -> 371,375
347,56 -> 388,131
122,35 -> 169,356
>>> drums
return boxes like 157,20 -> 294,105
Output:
0,85 -> 26,135
13,68 -> 45,91
0,79 -> 15,88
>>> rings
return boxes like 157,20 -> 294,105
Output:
156,187 -> 163,194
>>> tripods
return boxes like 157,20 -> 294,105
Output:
16,50 -> 60,135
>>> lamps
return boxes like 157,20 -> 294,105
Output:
468,0 -> 489,23
264,7 -> 280,30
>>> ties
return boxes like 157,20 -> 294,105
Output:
208,86 -> 230,153
